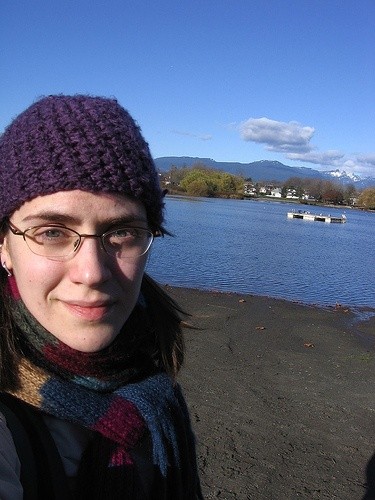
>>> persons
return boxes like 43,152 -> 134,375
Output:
0,93 -> 205,500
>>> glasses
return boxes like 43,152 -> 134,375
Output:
3,221 -> 157,259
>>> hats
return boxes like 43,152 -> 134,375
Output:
0,94 -> 168,223
